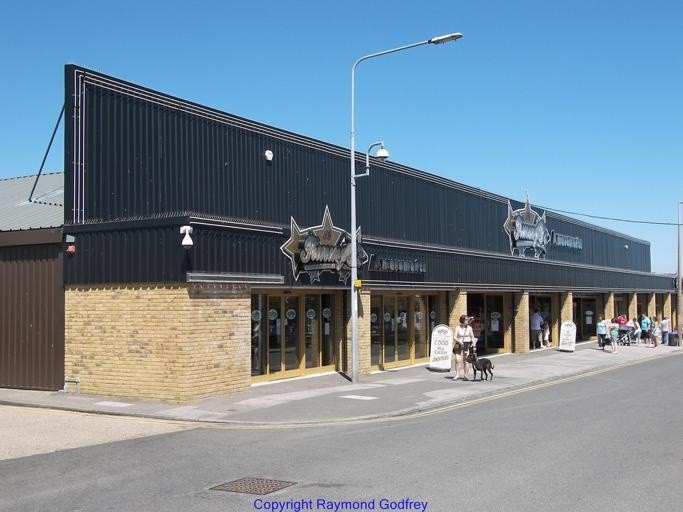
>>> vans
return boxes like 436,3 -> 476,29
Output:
371,305 -> 419,344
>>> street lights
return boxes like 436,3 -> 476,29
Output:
347,28 -> 467,386
677,200 -> 683,348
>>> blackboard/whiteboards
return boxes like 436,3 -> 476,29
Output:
559,321 -> 576,351
429,324 -> 453,369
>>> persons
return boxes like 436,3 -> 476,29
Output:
540,317 -> 552,348
449,314 -> 477,381
530,308 -> 546,350
250,319 -> 261,370
594,312 -> 670,354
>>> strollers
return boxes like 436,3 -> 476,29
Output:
606,326 -> 635,346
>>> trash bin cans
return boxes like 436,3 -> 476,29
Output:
668,332 -> 678,346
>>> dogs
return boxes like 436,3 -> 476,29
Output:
465,353 -> 496,381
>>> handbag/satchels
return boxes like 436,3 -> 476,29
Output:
453,343 -> 462,354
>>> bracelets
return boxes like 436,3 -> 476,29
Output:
460,343 -> 463,345
